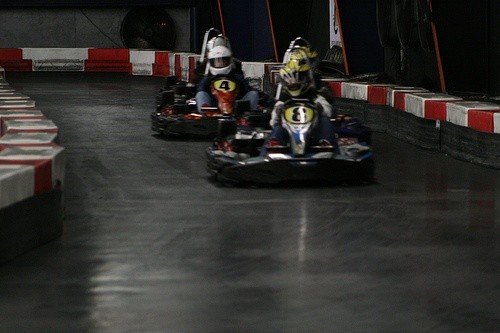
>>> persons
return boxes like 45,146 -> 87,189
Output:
192,45 -> 263,121
266,46 -> 337,132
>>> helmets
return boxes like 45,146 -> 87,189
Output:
207,34 -> 231,55
288,48 -> 311,65
279,59 -> 310,97
207,46 -> 233,76
289,37 -> 318,58
